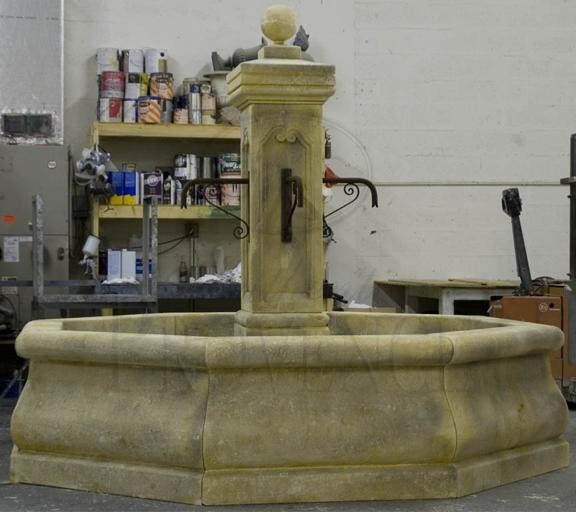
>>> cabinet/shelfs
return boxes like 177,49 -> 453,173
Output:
86,115 -> 247,295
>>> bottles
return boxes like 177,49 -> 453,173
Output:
179,255 -> 189,283
159,52 -> 167,72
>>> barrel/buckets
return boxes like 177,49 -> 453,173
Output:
174,152 -> 198,181
96,46 -> 175,124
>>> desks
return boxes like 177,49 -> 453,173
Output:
371,271 -> 545,333
